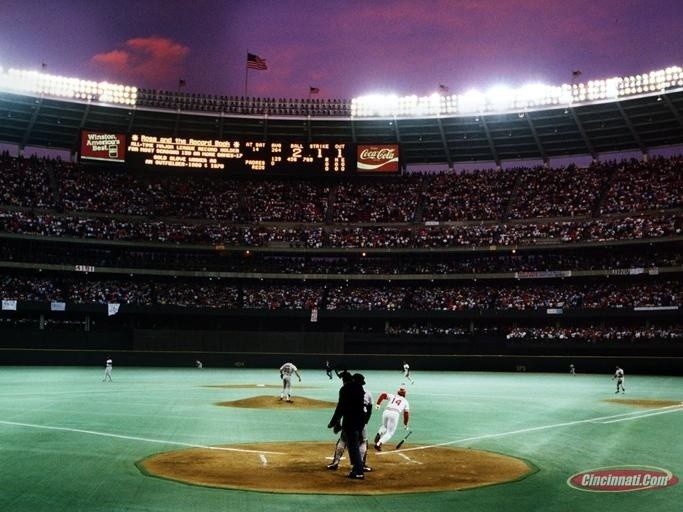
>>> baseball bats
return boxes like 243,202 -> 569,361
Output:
396,431 -> 412,450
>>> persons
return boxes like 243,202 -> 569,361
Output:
101,352 -> 113,382
278,358 -> 301,403
611,363 -> 625,394
321,357 -> 331,378
326,369 -> 364,480
193,360 -> 203,369
399,359 -> 415,385
374,387 -> 409,451
569,360 -> 576,376
326,373 -> 373,472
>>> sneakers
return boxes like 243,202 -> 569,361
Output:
326,461 -> 339,470
362,465 -> 373,472
345,472 -> 365,480
374,444 -> 382,452
374,432 -> 381,443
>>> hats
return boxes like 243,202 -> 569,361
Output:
397,387 -> 407,396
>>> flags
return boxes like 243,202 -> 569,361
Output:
572,70 -> 581,78
179,81 -> 184,87
438,84 -> 447,92
246,53 -> 267,71
309,87 -> 319,94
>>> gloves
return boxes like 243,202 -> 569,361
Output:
375,403 -> 381,411
403,424 -> 410,432
